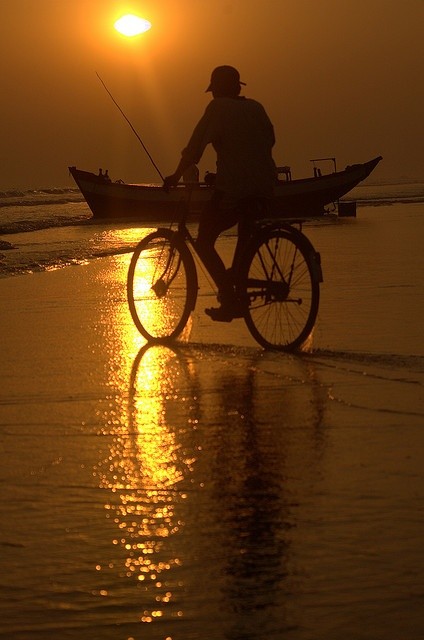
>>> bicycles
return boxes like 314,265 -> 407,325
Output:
127,173 -> 322,349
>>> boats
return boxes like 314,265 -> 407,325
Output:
69,161 -> 383,218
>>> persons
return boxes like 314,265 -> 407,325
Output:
161,63 -> 278,324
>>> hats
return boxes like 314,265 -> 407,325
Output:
204,65 -> 246,92
